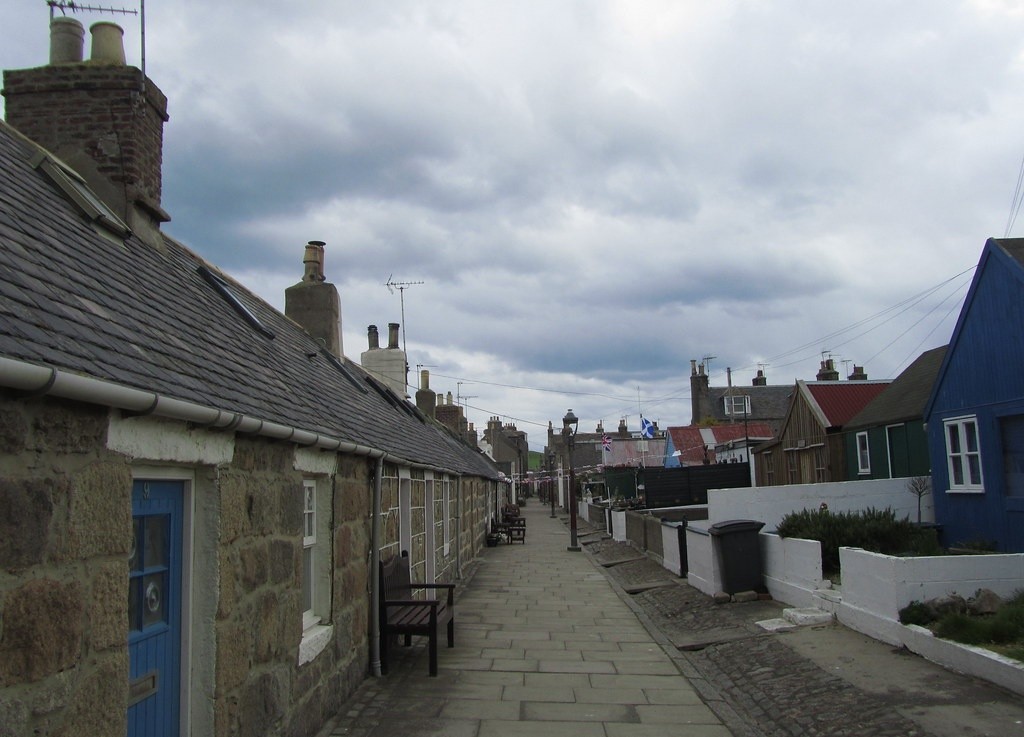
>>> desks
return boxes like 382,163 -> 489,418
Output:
509,526 -> 526,544
511,517 -> 527,527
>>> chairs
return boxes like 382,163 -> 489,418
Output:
378,549 -> 456,677
485,502 -> 520,545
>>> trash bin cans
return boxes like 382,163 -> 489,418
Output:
707,518 -> 766,594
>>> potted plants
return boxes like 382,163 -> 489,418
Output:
612,499 -> 637,512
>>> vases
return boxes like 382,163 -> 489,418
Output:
487,539 -> 498,548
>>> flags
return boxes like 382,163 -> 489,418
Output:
602,433 -> 612,451
641,417 -> 654,439
